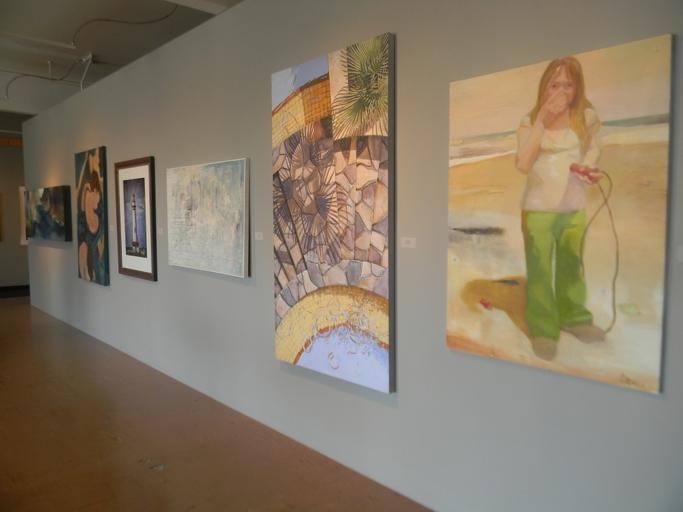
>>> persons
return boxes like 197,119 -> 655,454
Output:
514,58 -> 607,363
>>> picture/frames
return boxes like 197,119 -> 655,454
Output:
114,156 -> 157,282
